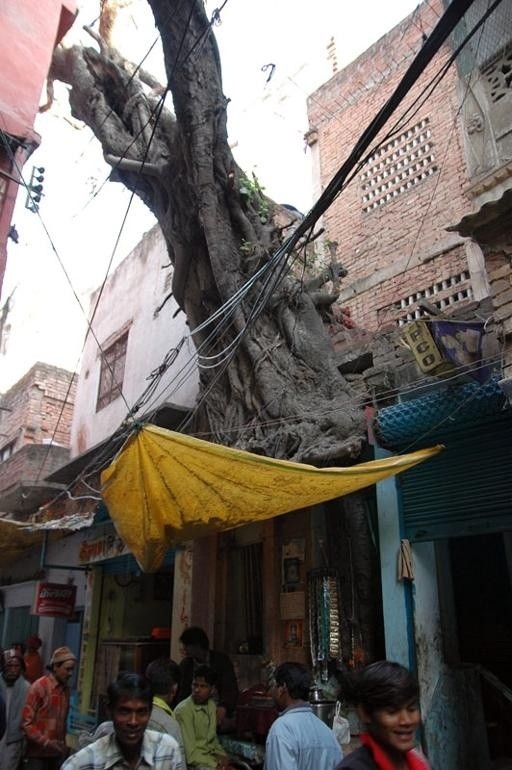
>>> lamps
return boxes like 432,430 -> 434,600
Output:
25,163 -> 47,213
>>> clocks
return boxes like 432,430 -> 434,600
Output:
114,572 -> 133,588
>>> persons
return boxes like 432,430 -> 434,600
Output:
1,634 -> 234,769
175,626 -> 240,695
264,661 -> 343,769
335,660 -> 433,770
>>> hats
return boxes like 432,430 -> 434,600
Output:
1,650 -> 25,669
24,637 -> 42,648
48,647 -> 76,667
